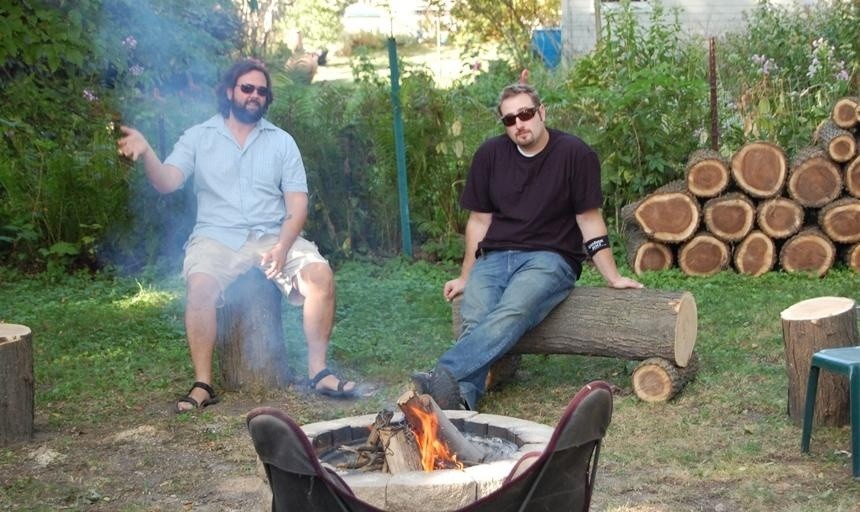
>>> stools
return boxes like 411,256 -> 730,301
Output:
801,346 -> 859,477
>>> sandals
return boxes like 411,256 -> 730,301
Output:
409,369 -> 462,409
175,383 -> 218,413
307,369 -> 357,397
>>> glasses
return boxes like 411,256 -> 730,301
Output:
501,107 -> 539,125
235,83 -> 268,95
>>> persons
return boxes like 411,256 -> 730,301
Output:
410,85 -> 644,410
116,59 -> 357,414
